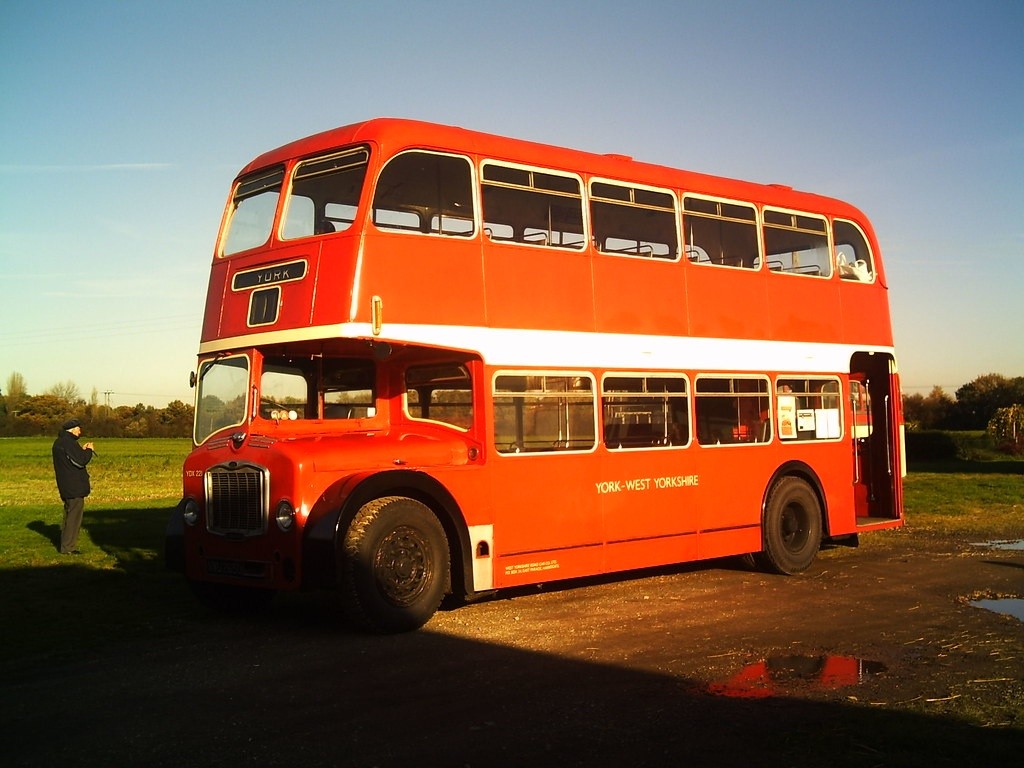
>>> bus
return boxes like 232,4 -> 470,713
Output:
172,119 -> 905,631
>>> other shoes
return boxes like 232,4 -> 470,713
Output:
67,550 -> 82,554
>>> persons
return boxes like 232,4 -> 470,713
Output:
52,420 -> 94,555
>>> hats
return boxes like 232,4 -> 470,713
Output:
63,419 -> 84,430
